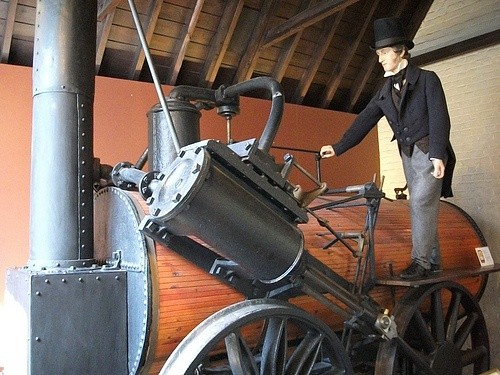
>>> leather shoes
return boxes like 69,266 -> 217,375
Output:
400,263 -> 431,278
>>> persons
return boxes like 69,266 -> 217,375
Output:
320,15 -> 456,281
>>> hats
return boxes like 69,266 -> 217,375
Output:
369,18 -> 415,52
